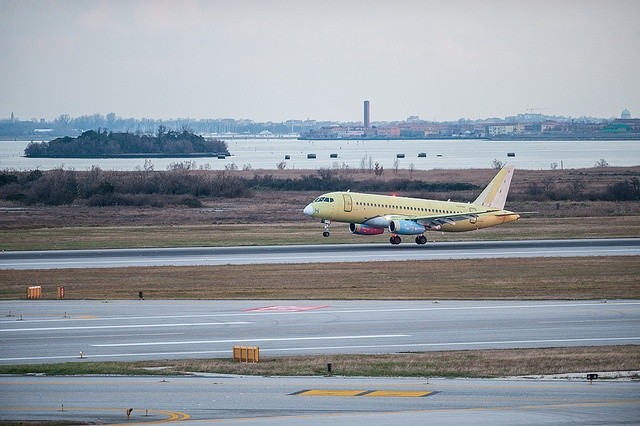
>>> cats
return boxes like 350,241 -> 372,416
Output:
303,161 -> 539,244
